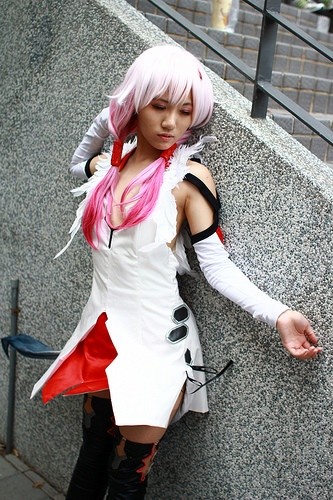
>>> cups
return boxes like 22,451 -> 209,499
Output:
212,0 -> 232,30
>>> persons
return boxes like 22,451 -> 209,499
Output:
30,44 -> 326,499
